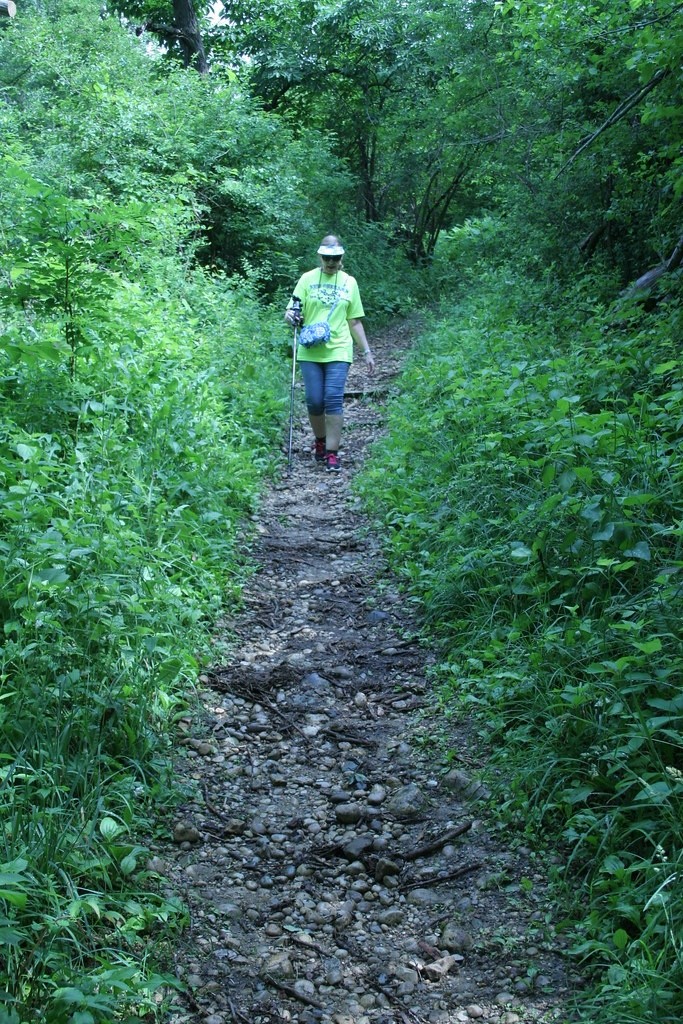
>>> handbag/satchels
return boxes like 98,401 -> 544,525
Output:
299,322 -> 330,349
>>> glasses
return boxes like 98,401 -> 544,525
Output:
321,255 -> 342,262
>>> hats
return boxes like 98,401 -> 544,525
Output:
317,235 -> 345,255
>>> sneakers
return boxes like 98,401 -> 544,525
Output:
324,453 -> 341,472
315,439 -> 327,464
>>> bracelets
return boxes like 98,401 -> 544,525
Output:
363,350 -> 371,356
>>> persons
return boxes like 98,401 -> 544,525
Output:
283,235 -> 376,472
